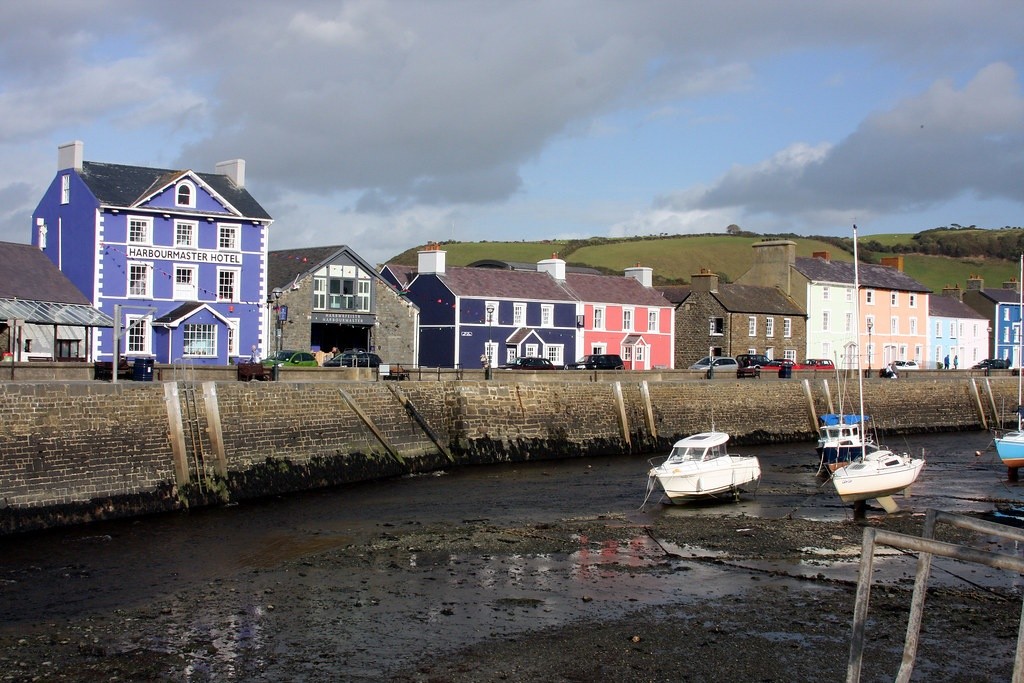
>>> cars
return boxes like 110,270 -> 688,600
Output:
688,353 -> 835,372
497,356 -> 556,370
260,349 -> 319,369
885,360 -> 920,369
323,352 -> 384,367
972,358 -> 1005,369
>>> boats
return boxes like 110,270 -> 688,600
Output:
646,421 -> 761,505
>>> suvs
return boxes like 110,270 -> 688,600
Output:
564,354 -> 626,370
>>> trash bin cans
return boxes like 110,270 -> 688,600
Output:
985,369 -> 988,376
865,369 -> 869,378
485,369 -> 492,380
133,357 -> 154,381
780,364 -> 792,378
271,366 -> 280,381
1014,369 -> 1019,375
706,369 -> 711,378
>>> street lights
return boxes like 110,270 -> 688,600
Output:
709,315 -> 715,379
271,287 -> 284,381
867,319 -> 873,378
486,305 -> 495,380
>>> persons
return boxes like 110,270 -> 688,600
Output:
1006,357 -> 1011,369
738,358 -> 760,375
944,355 -> 950,370
885,362 -> 898,378
249,345 -> 262,364
954,355 -> 958,369
480,354 -> 487,370
332,347 -> 340,360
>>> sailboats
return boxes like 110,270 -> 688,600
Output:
992,252 -> 1024,468
814,223 -> 926,503
